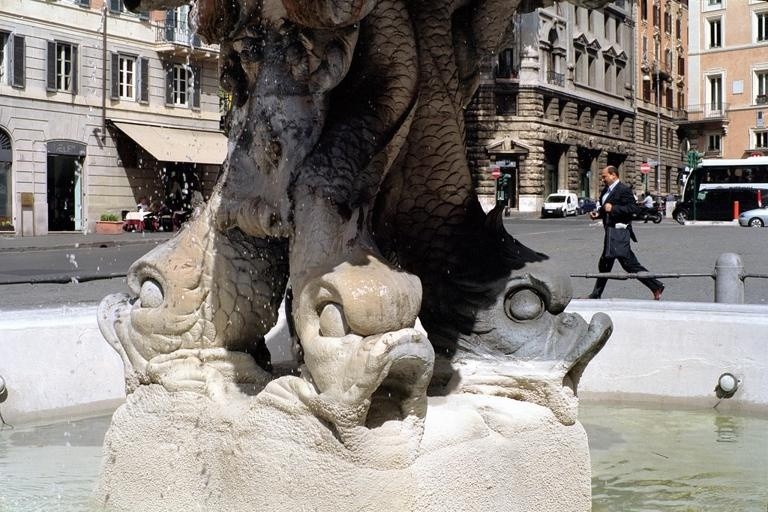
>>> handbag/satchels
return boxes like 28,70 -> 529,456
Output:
604,227 -> 630,259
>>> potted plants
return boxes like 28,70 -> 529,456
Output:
94,213 -> 124,235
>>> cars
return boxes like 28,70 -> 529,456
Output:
578,197 -> 596,215
738,205 -> 768,229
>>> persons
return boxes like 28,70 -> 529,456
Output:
135,176 -> 183,233
577,165 -> 665,300
637,191 -> 653,223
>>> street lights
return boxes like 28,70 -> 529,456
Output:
638,42 -> 661,206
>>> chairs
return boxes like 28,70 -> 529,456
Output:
119,207 -> 191,233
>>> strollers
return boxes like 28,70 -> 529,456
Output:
652,196 -> 666,216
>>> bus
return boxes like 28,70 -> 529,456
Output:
673,155 -> 767,208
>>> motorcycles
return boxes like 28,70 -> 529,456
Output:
634,202 -> 663,223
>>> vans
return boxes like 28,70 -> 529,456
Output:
542,192 -> 580,218
671,189 -> 759,225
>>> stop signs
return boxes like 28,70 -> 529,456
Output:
492,168 -> 500,178
640,163 -> 650,174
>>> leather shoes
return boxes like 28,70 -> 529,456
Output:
653,283 -> 664,301
